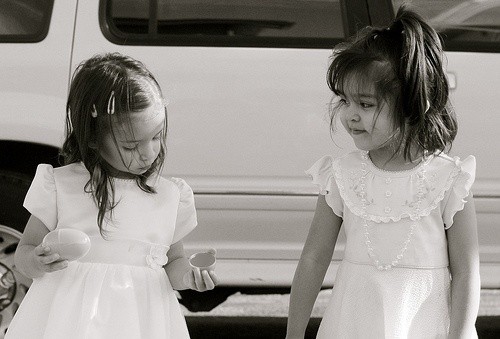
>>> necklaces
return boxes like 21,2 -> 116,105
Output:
358,151 -> 428,271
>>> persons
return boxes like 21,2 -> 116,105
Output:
284,3 -> 481,339
3,52 -> 222,339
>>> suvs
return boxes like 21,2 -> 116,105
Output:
0,1 -> 500,339
